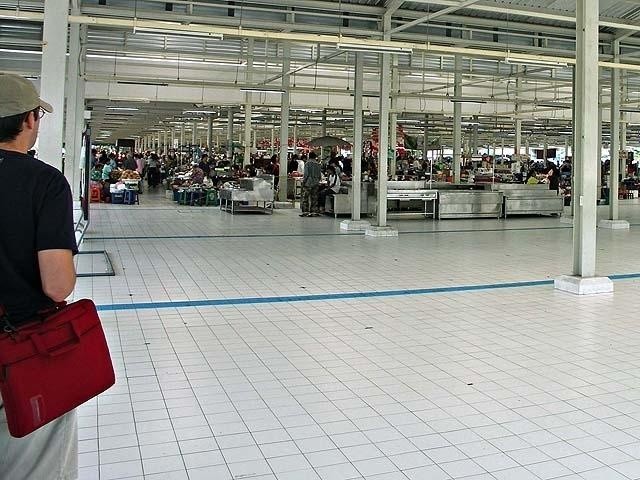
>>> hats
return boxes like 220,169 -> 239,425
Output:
0,73 -> 54,118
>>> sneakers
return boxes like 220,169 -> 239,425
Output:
298,213 -> 318,217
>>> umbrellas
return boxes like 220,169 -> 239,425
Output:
304,136 -> 353,156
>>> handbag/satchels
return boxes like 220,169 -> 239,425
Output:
0,298 -> 115,437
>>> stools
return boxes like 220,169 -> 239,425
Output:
89,186 -> 143,206
178,184 -> 218,208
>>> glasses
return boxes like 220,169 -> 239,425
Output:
23,107 -> 46,122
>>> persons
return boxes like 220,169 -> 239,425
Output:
0,71 -> 78,480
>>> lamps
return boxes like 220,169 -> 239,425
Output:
504,54 -> 573,69
132,23 -> 224,44
334,39 -> 415,58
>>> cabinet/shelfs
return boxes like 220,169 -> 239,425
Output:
326,178 -> 565,224
225,172 -> 278,216
